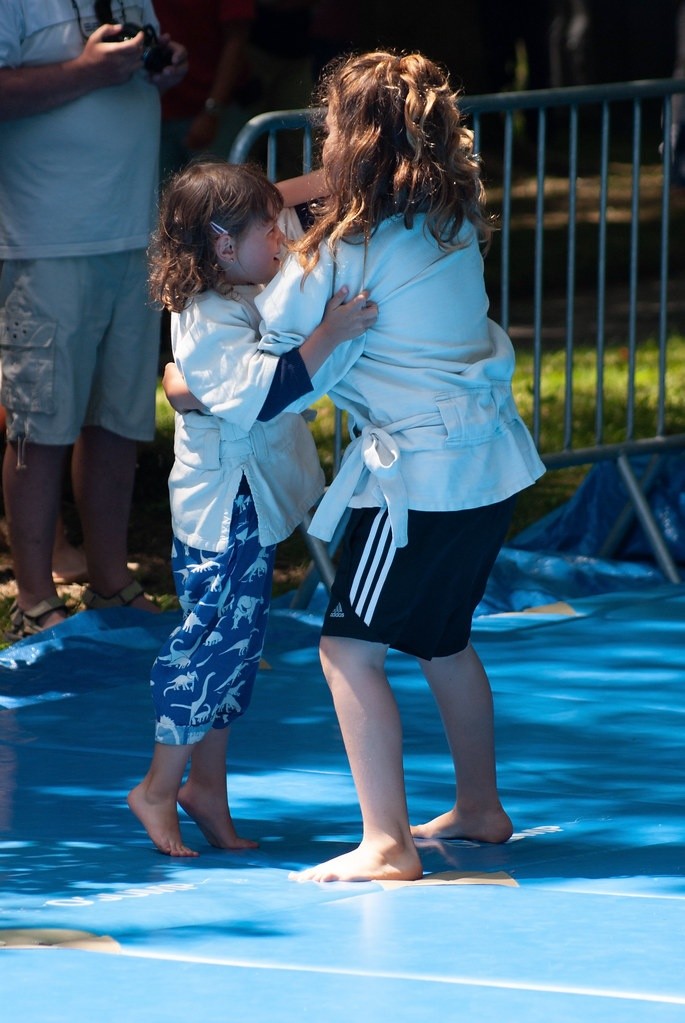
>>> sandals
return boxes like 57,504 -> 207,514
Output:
5,594 -> 65,640
76,579 -> 145,610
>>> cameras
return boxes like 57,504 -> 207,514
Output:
103,22 -> 171,72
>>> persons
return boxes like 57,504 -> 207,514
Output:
128,163 -> 378,856
161,51 -> 546,882
0,0 -> 182,641
